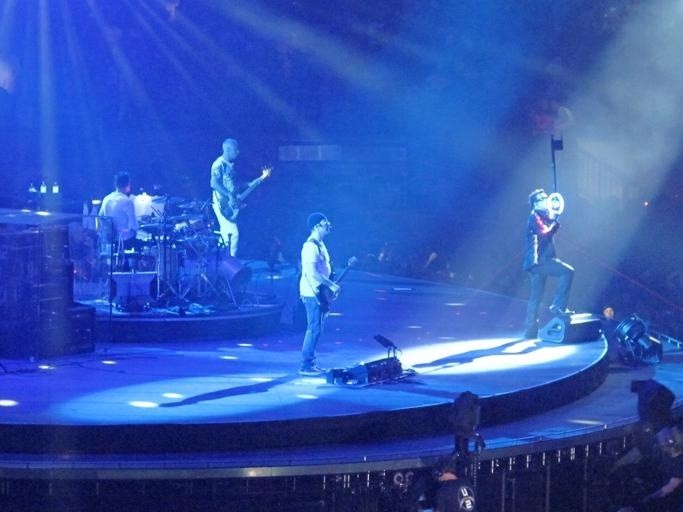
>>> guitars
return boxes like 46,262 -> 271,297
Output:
322,256 -> 358,302
220,165 -> 274,220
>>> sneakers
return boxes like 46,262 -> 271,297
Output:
299,367 -> 326,375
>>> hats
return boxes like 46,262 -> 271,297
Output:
307,214 -> 325,230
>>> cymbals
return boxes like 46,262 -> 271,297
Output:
178,201 -> 213,208
141,224 -> 174,231
151,197 -> 184,203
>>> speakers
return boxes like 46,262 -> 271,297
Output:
0,226 -> 95,359
349,357 -> 402,385
538,313 -> 603,343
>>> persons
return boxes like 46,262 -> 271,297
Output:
522,188 -> 574,338
615,418 -> 683,512
577,250 -> 683,340
529,95 -> 551,136
551,99 -> 580,137
296,213 -> 340,375
432,460 -> 476,512
98,171 -> 149,251
211,138 -> 264,257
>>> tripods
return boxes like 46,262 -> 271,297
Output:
158,215 -> 239,311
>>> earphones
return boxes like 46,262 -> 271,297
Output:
316,223 -> 321,228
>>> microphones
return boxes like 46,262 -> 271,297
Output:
199,198 -> 210,213
329,227 -> 335,232
